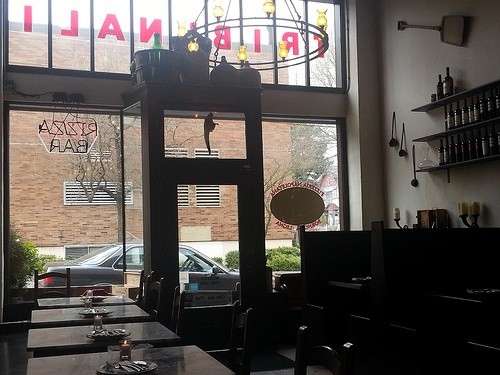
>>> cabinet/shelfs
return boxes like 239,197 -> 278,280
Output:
411,79 -> 500,183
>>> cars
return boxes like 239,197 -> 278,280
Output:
38,241 -> 241,303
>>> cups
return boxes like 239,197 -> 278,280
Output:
431,94 -> 436,102
87,290 -> 93,296
94,316 -> 104,331
84,297 -> 93,309
119,339 -> 132,359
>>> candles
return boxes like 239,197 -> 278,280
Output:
85,299 -> 93,309
393,208 -> 400,219
458,201 -> 468,215
120,340 -> 131,361
87,290 -> 93,297
94,315 -> 103,329
470,201 -> 480,214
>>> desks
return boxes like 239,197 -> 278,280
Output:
26,345 -> 236,375
30,305 -> 151,329
26,322 -> 181,358
37,296 -> 136,309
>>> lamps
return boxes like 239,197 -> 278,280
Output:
177,0 -> 330,71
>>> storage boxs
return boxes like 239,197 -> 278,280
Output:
111,271 -> 141,299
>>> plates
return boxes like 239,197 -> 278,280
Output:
466,288 -> 500,294
78,308 -> 114,317
86,330 -> 132,340
80,297 -> 106,301
352,277 -> 372,283
96,360 -> 158,375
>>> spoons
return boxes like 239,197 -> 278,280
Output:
113,362 -> 131,373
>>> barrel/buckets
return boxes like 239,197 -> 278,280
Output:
171,29 -> 212,87
131,49 -> 182,84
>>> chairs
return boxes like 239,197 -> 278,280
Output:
137,270 -> 356,375
34,268 -> 70,307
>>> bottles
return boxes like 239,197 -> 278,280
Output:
497,130 -> 500,154
436,67 -> 500,130
454,134 -> 461,164
210,54 -> 262,86
449,136 -> 455,164
488,123 -> 499,156
482,125 -> 490,158
149,32 -> 162,49
467,130 -> 475,161
475,127 -> 482,159
444,136 -> 449,166
438,138 -> 444,167
460,132 -> 468,162
